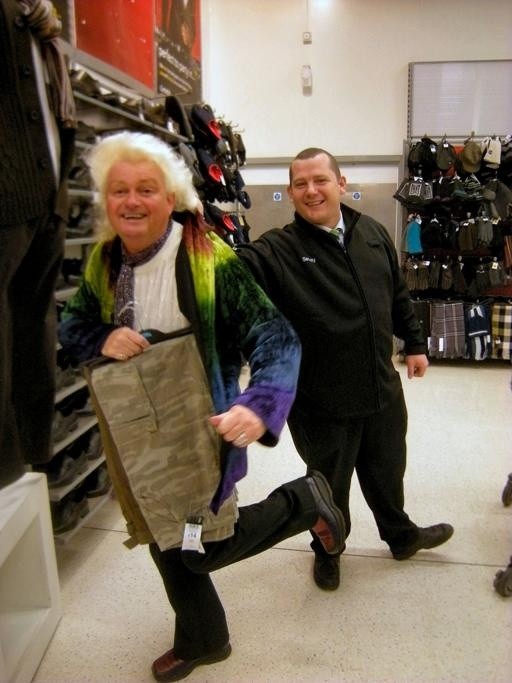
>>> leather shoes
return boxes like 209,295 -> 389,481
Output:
51,350 -> 112,533
391,523 -> 455,561
151,640 -> 233,683
304,467 -> 349,592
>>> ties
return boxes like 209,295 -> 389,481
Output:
331,227 -> 340,239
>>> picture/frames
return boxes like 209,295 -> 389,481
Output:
67,1 -> 159,100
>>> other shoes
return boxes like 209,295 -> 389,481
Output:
57,165 -> 93,322
70,68 -> 164,144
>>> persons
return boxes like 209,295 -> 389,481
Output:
235,147 -> 454,592
58,130 -> 347,683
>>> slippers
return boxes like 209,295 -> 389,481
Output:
165,94 -> 250,251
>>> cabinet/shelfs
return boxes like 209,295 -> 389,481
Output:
0,472 -> 65,682
45,90 -> 190,543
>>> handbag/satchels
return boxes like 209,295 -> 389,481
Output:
408,297 -> 512,361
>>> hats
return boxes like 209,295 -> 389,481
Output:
461,142 -> 481,173
436,137 -> 456,170
